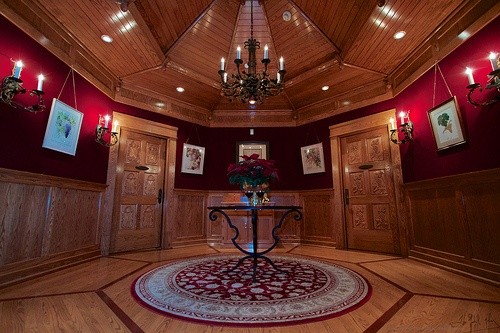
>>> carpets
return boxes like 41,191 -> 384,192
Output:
129,256 -> 373,328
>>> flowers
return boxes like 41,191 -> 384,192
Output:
225,154 -> 277,186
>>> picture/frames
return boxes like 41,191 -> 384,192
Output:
427,96 -> 467,152
300,142 -> 326,175
236,141 -> 270,164
42,98 -> 84,156
180,143 -> 205,175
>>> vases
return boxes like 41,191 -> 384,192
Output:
243,183 -> 267,192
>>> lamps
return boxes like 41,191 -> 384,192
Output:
217,0 -> 288,104
377,0 -> 386,7
117,0 -> 130,12
465,50 -> 500,107
95,114 -> 120,147
0,57 -> 46,113
388,111 -> 413,144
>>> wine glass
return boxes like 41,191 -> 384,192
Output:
243,185 -> 255,206
255,185 -> 265,206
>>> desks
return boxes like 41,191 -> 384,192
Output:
207,205 -> 303,283
